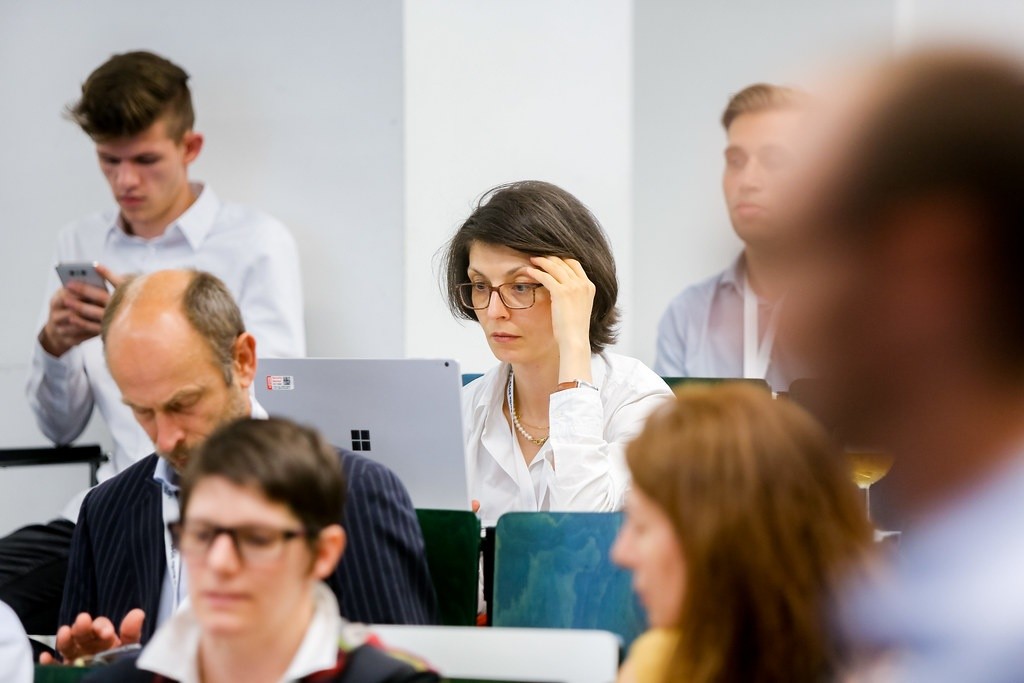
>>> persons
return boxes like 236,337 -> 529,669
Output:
650,86 -> 831,396
0,53 -> 307,635
37,267 -> 442,665
441,179 -> 679,613
87,417 -> 445,683
604,382 -> 875,682
750,42 -> 1023,682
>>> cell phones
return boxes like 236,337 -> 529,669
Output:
56,262 -> 109,295
94,644 -> 142,666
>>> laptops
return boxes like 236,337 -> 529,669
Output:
253,358 -> 472,513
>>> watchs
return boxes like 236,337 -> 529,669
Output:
556,379 -> 599,392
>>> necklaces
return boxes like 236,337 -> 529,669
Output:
511,407 -> 550,446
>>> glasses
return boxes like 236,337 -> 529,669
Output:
455,282 -> 543,310
167,521 -> 308,559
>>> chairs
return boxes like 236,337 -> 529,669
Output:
349,506 -> 652,683
